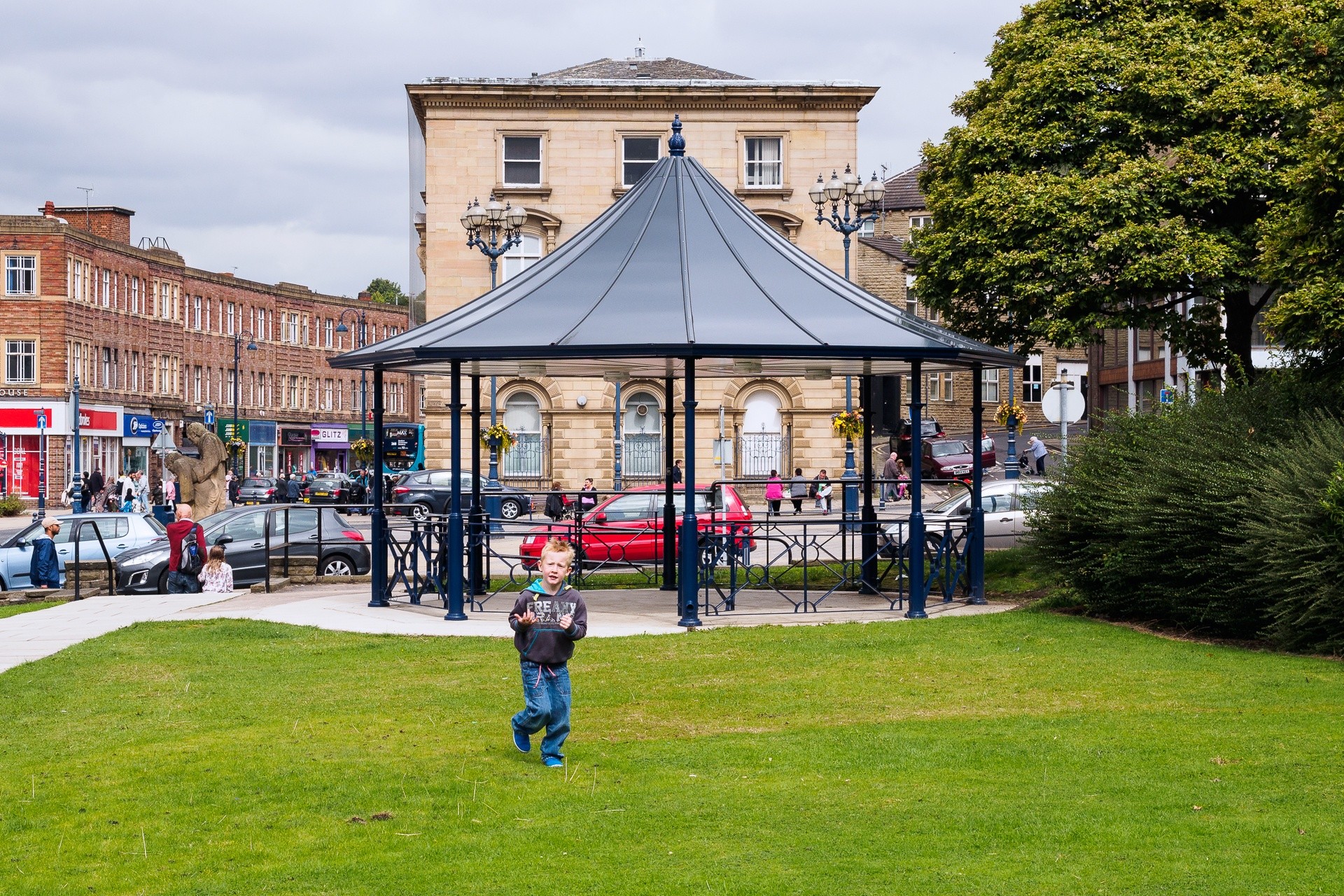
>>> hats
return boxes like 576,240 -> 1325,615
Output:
95,467 -> 101,470
41,517 -> 64,528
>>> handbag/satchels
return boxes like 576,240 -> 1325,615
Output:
82,483 -> 89,491
234,500 -> 245,507
179,523 -> 203,574
168,499 -> 174,510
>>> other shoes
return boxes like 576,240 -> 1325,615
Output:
793,513 -> 796,515
774,513 -> 780,516
823,510 -> 827,515
816,495 -> 821,501
886,500 -> 892,503
986,472 -> 989,475
983,472 -> 986,476
799,510 -> 802,514
894,499 -> 898,501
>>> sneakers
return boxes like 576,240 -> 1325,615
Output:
513,730 -> 530,753
542,756 -> 563,768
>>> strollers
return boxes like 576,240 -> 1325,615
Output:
1020,451 -> 1035,475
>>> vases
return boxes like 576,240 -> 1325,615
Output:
948,487 -> 964,496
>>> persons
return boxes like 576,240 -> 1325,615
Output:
673,460 -> 683,483
508,540 -> 588,768
1025,436 -> 1049,476
973,429 -> 992,477
810,470 -> 833,515
31,463 -> 424,592
766,470 -> 784,516
789,468 -> 807,515
547,478 -> 598,522
884,453 -> 907,503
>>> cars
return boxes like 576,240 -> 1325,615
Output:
923,437 -> 997,484
285,474 -> 317,502
1,512 -> 169,592
111,502 -> 370,595
315,467 -> 414,503
303,479 -> 352,512
237,476 -> 280,504
519,483 -> 758,567
391,469 -> 530,522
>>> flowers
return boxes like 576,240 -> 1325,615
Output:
226,437 -> 246,459
948,477 -> 973,488
480,422 -> 518,463
830,407 -> 864,442
351,437 -> 374,464
993,398 -> 1028,437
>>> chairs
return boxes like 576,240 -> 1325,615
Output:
242,515 -> 257,538
952,449 -> 959,453
81,525 -> 96,540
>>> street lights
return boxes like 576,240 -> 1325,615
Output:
231,330 -> 258,508
809,167 -> 889,540
335,307 -> 366,471
458,189 -> 530,537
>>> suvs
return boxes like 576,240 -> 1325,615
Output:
889,417 -> 947,464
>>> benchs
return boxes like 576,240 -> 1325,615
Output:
766,497 -> 833,515
531,495 -> 579,511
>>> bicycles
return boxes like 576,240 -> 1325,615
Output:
551,499 -> 578,522
